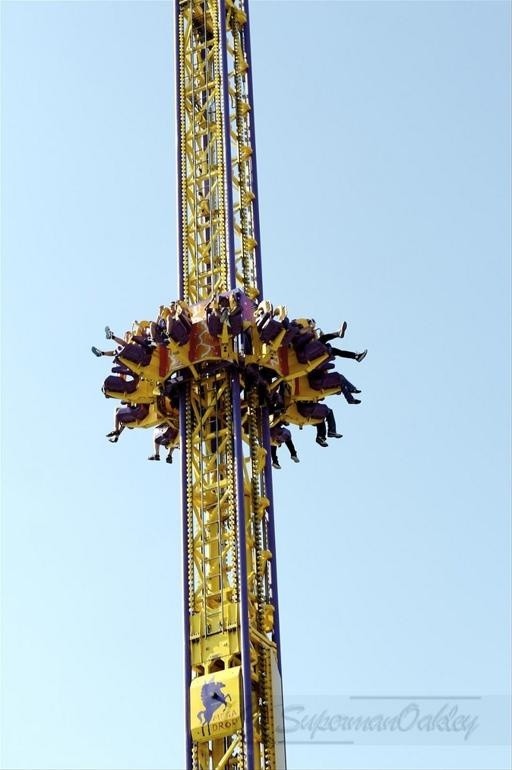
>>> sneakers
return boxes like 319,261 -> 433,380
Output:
105,325 -> 114,340
356,350 -> 368,362
92,346 -> 103,357
148,455 -> 160,461
106,431 -> 119,442
349,388 -> 361,404
339,321 -> 347,338
166,455 -> 172,463
315,430 -> 343,447
144,332 -> 172,342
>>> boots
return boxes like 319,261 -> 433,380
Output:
291,451 -> 300,463
272,456 -> 281,469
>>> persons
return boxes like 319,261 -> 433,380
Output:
90,292 -> 369,471
